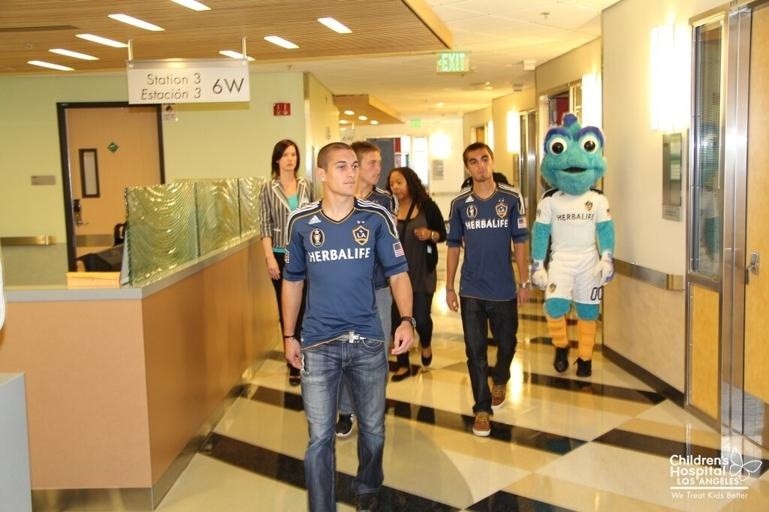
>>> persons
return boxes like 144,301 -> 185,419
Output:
384,166 -> 446,381
333,138 -> 401,437
282,141 -> 417,512
446,142 -> 531,438
531,109 -> 615,377
257,137 -> 310,385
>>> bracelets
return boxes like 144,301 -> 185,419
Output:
430,230 -> 436,239
519,279 -> 530,289
447,288 -> 455,293
282,334 -> 296,342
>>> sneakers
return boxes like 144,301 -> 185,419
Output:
574,358 -> 591,376
471,411 -> 493,437
555,348 -> 568,372
356,493 -> 380,512
335,413 -> 352,437
491,384 -> 507,410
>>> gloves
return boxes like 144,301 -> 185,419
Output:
533,269 -> 549,292
593,260 -> 614,288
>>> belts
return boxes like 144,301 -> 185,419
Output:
300,331 -> 367,352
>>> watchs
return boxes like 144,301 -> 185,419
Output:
399,316 -> 417,328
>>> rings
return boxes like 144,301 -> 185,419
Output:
422,231 -> 424,235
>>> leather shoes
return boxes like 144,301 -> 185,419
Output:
391,368 -> 411,382
289,374 -> 301,387
421,349 -> 432,365
